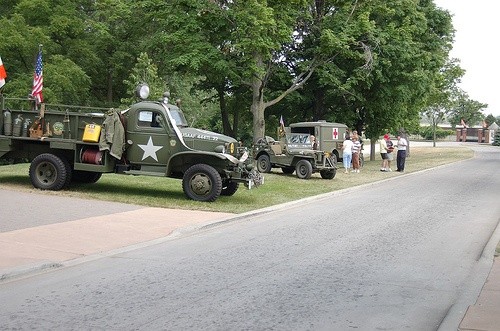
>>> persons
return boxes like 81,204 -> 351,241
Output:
350,137 -> 362,173
350,130 -> 364,168
380,134 -> 393,172
342,135 -> 354,174
394,134 -> 407,172
384,136 -> 394,172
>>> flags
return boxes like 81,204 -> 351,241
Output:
0,56 -> 7,92
31,49 -> 44,103
